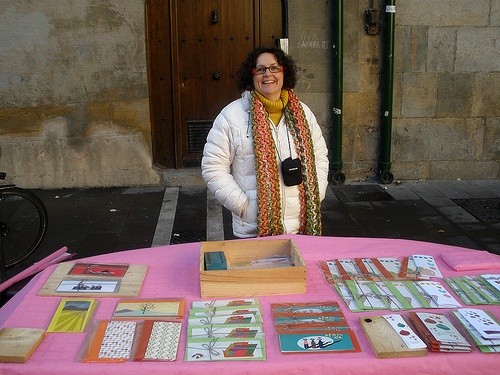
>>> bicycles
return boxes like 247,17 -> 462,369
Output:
0,169 -> 48,271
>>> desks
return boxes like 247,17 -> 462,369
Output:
1,234 -> 500,375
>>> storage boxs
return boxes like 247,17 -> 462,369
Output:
199,238 -> 307,298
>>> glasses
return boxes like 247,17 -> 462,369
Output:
251,66 -> 288,75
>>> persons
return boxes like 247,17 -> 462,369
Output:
202,45 -> 329,241
